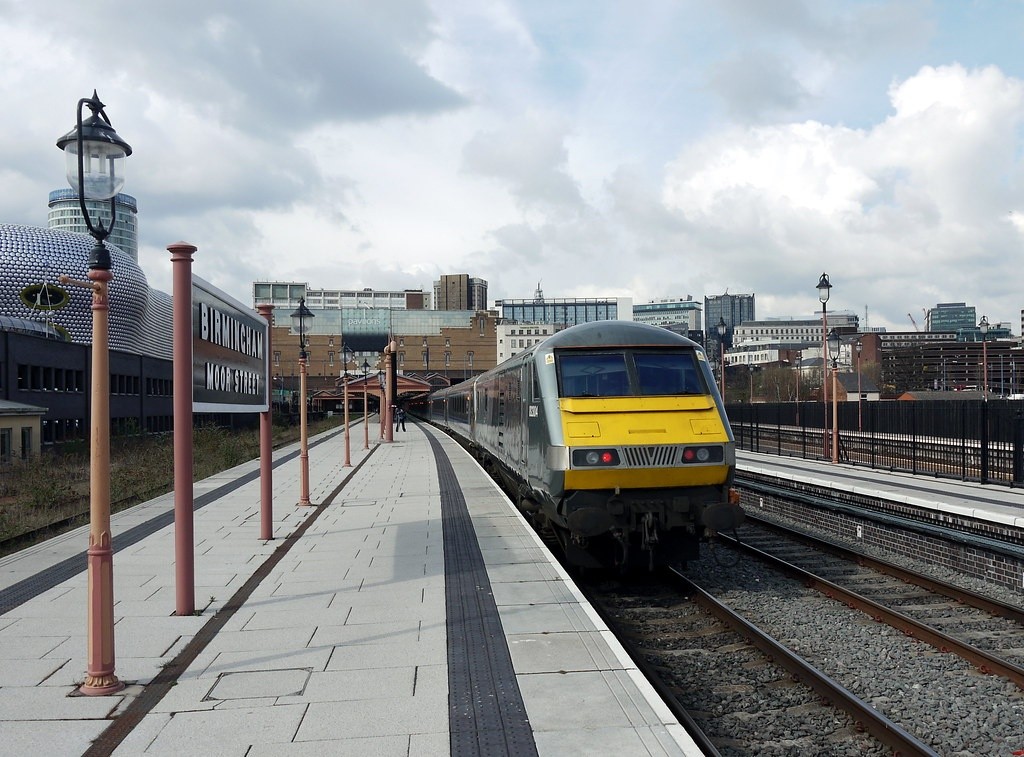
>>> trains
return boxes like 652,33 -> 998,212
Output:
403,319 -> 744,572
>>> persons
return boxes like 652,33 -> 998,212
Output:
396,407 -> 406,431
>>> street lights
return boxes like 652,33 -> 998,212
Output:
825,325 -> 843,464
56,89 -> 135,698
746,363 -> 757,403
377,368 -> 387,439
855,337 -> 863,432
795,354 -> 800,426
816,273 -> 833,461
978,314 -> 989,403
338,341 -> 354,468
716,308 -> 728,406
361,359 -> 370,450
289,294 -> 316,506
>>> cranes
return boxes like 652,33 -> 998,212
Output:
908,308 -> 927,332
